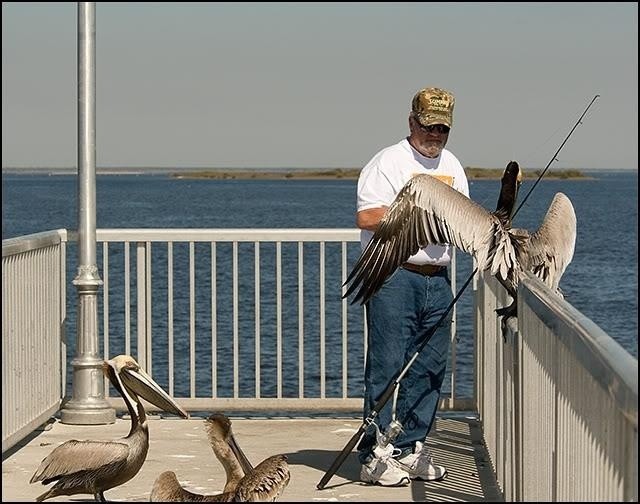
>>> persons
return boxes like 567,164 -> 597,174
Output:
356,87 -> 470,488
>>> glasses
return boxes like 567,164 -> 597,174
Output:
413,115 -> 452,135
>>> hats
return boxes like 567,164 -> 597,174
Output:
410,87 -> 456,130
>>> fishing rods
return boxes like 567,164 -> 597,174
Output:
316,95 -> 601,490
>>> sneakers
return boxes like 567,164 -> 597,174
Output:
398,441 -> 449,482
359,440 -> 412,489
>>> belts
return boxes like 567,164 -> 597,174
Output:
404,261 -> 448,278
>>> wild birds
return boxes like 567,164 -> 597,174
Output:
338,159 -> 579,345
148,410 -> 291,502
29,353 -> 191,503
